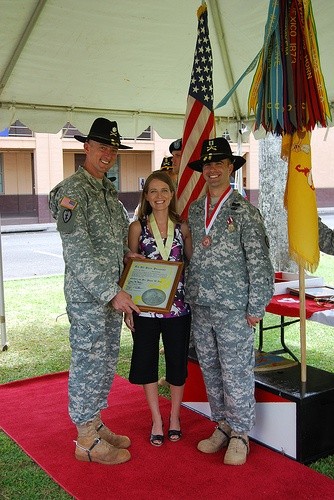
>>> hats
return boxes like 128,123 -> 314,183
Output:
74,118 -> 133,150
169,138 -> 184,154
161,157 -> 174,171
188,138 -> 246,172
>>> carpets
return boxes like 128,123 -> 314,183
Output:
0,369 -> 334,500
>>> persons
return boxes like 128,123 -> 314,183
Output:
185,137 -> 274,466
124,172 -> 194,446
156,138 -> 183,388
49,116 -> 134,465
317,216 -> 334,256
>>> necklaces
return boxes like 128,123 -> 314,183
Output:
161,230 -> 166,239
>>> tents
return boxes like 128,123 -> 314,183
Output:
1,0 -> 334,353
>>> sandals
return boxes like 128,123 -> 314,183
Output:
150,420 -> 164,447
168,415 -> 181,442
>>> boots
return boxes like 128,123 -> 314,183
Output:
73,422 -> 131,465
94,413 -> 131,448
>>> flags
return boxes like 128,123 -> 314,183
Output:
278,128 -> 320,273
173,3 -> 216,220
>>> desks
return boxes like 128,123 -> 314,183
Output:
255,281 -> 334,363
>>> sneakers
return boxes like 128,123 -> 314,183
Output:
224,430 -> 250,466
197,421 -> 232,454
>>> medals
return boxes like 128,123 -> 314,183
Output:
202,236 -> 211,247
